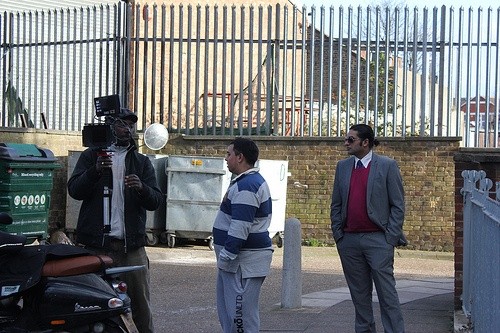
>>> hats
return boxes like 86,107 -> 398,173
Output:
106,108 -> 138,125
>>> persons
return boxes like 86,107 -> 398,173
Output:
330,123 -> 408,333
67,108 -> 163,333
212,138 -> 273,333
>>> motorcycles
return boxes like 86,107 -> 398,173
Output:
0,212 -> 148,333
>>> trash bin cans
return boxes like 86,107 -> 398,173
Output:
165,155 -> 232,251
254,159 -> 289,248
65,150 -> 82,241
141,154 -> 169,246
0,143 -> 61,244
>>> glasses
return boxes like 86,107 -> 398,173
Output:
345,136 -> 364,143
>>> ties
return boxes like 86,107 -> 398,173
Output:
356,160 -> 364,169
